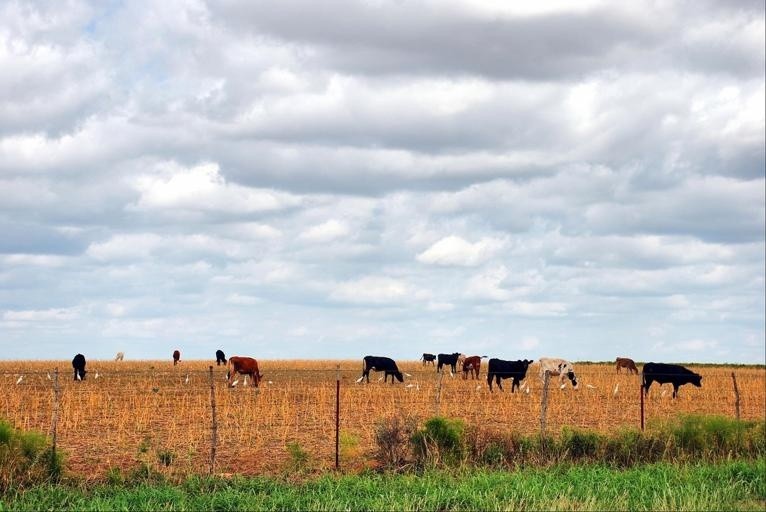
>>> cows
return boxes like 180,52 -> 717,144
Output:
537,358 -> 580,392
361,356 -> 404,385
72,354 -> 88,380
172,350 -> 180,366
616,356 -> 639,375
420,352 -> 481,382
642,362 -> 703,401
226,356 -> 264,389
487,358 -> 533,393
216,349 -> 227,366
